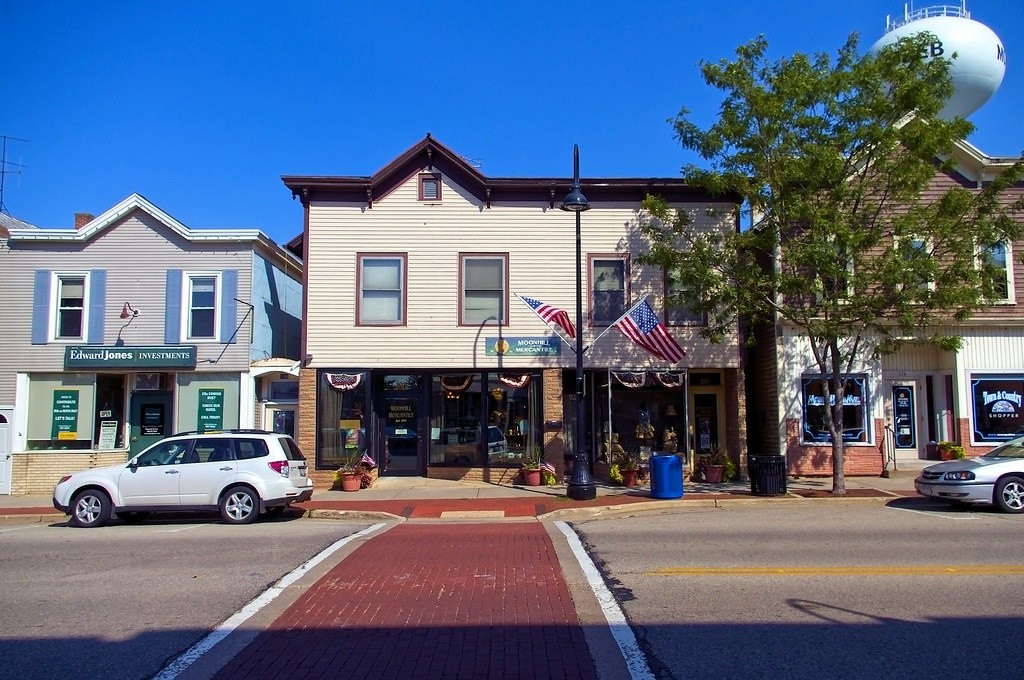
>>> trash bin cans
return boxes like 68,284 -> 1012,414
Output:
746,454 -> 788,497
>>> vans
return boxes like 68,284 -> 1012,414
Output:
430,426 -> 508,466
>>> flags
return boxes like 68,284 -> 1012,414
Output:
361,453 -> 376,468
614,298 -> 688,366
538,459 -> 557,476
514,293 -> 577,339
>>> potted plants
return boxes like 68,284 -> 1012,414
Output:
338,445 -> 365,491
522,442 -> 550,485
617,442 -> 640,487
699,443 -> 726,483
938,440 -> 966,461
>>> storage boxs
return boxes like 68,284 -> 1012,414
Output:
650,452 -> 684,499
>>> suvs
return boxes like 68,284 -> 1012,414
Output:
50,428 -> 314,529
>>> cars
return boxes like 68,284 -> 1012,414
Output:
912,429 -> 1023,516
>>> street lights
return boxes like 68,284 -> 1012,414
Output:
561,142 -> 602,498
